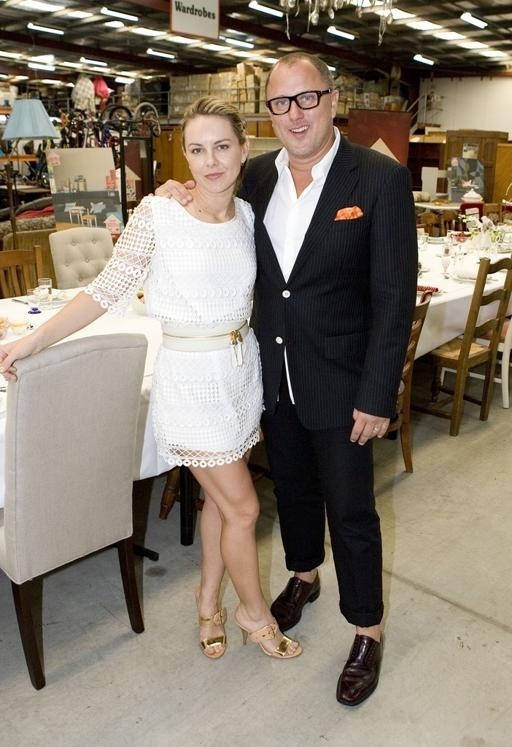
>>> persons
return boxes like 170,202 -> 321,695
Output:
1,94 -> 304,659
148,51 -> 419,709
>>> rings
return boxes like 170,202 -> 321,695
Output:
373,427 -> 381,432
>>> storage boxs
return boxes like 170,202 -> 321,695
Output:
168,62 -> 364,116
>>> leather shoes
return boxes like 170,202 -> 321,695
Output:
337,632 -> 383,706
270,571 -> 320,632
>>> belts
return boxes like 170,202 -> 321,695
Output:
162,320 -> 248,350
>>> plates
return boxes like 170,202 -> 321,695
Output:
419,215 -> 511,296
25,290 -> 74,309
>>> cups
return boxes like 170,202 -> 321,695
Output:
10,315 -> 30,335
36,276 -> 52,310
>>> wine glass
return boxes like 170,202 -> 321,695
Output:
27,286 -> 42,315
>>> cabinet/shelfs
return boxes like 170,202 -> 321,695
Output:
151,121 -> 276,192
442,129 -> 508,204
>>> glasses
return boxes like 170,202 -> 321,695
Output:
265,89 -> 331,115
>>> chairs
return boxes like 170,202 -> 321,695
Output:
0,333 -> 148,690
49,226 -> 114,289
158,466 -> 206,544
387,192 -> 512,474
0,245 -> 44,298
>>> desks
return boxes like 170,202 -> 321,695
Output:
0,184 -> 50,196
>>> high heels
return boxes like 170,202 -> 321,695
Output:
234,606 -> 303,659
196,591 -> 227,658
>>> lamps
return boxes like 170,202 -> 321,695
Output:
2,99 -> 61,251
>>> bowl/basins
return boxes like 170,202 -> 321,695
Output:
461,196 -> 484,203
